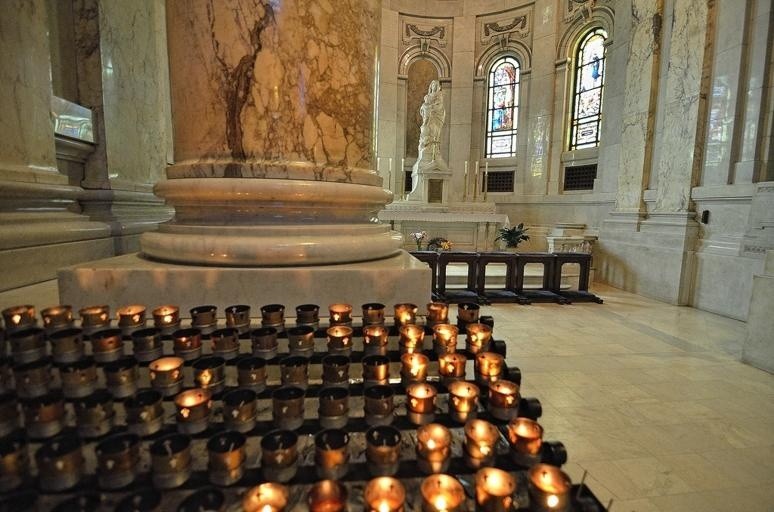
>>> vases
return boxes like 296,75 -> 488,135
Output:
416,241 -> 422,251
429,245 -> 443,251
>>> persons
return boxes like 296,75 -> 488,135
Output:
419,95 -> 431,125
415,79 -> 450,171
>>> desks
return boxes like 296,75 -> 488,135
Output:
410,251 -> 603,306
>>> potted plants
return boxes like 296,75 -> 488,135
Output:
494,221 -> 531,254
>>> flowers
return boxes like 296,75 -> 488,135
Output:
409,230 -> 427,244
427,238 -> 453,252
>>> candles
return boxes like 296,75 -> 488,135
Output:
475,161 -> 478,174
376,157 -> 380,171
389,157 -> 392,171
401,158 -> 404,171
485,161 -> 488,176
464,160 -> 468,175
